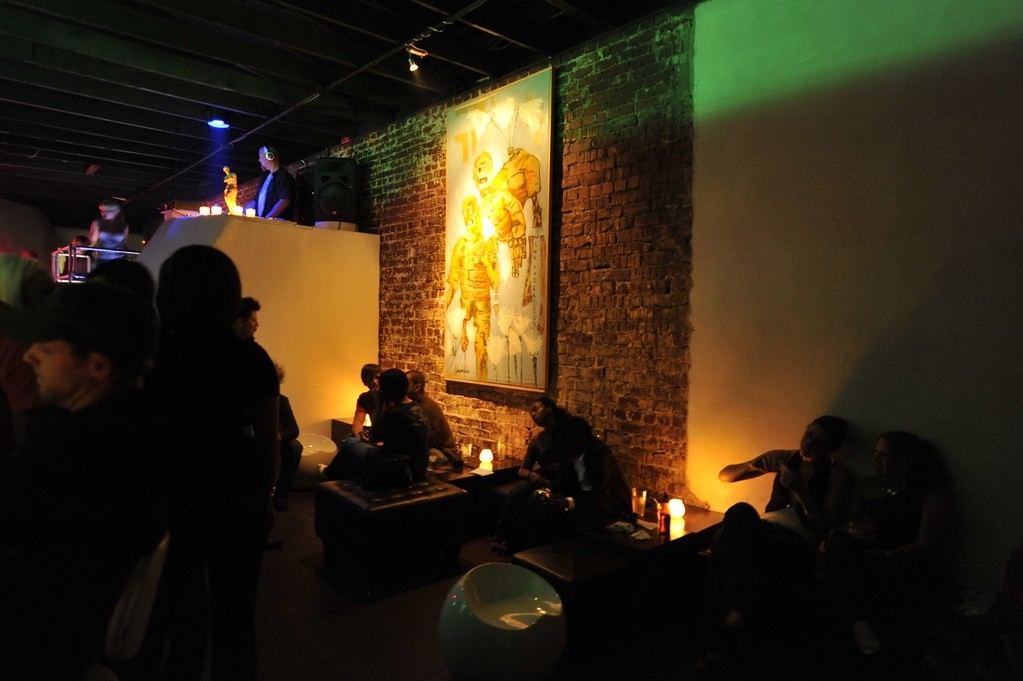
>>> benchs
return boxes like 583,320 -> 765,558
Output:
489,475 -> 525,496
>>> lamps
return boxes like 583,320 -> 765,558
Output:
479,449 -> 493,473
207,106 -> 232,129
668,498 -> 684,541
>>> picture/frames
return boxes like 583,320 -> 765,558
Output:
444,67 -> 552,393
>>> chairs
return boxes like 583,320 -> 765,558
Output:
969,543 -> 1023,631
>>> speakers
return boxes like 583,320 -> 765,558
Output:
296,157 -> 359,230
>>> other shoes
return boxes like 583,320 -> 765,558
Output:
317,462 -> 328,476
491,540 -> 523,554
276,498 -> 288,512
264,535 -> 281,550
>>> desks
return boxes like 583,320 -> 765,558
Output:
425,452 -> 523,485
575,499 -> 726,604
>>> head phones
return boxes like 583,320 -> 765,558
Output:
262,145 -> 275,161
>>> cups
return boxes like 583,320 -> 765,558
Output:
497,441 -> 505,460
461,443 -> 471,463
631,487 -> 647,516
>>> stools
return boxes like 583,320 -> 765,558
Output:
315,473 -> 471,598
512,537 -> 634,655
437,561 -> 567,681
297,432 -> 338,474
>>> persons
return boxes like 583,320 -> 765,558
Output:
317,364 -> 458,493
1,244 -> 302,681
714,416 -> 943,655
58,199 -> 128,281
254,144 -> 295,221
489,397 -> 632,556
222,165 -> 243,216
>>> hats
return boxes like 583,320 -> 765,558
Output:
0,283 -> 147,372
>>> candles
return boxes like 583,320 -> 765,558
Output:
233,205 -> 243,216
246,208 -> 255,217
200,206 -> 210,216
212,206 -> 222,215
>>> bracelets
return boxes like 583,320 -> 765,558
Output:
565,497 -> 575,511
528,471 -> 531,477
